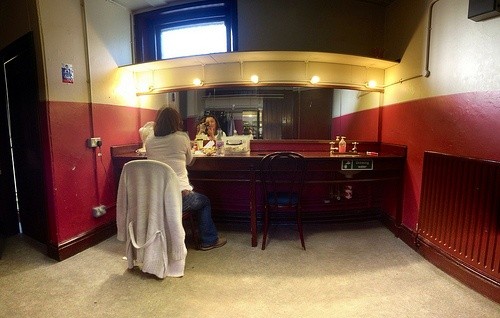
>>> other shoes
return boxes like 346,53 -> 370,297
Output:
199,240 -> 228,251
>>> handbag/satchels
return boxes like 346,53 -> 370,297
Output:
223,139 -> 251,153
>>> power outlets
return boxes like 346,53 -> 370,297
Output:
88,136 -> 101,148
93,204 -> 106,218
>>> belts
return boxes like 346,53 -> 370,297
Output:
182,190 -> 190,198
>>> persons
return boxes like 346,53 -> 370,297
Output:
194,114 -> 226,141
145,105 -> 228,251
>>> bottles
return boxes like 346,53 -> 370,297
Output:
215,128 -> 224,157
197,139 -> 203,150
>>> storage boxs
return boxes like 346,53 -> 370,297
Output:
221,135 -> 253,152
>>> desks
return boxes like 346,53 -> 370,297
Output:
113,150 -> 406,246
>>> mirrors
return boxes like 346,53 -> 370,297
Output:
140,84 -> 383,142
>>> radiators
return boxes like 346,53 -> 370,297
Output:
418,151 -> 500,285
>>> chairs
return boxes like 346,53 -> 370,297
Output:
116,160 -> 200,279
259,152 -> 307,250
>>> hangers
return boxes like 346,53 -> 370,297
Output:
209,109 -> 233,118
